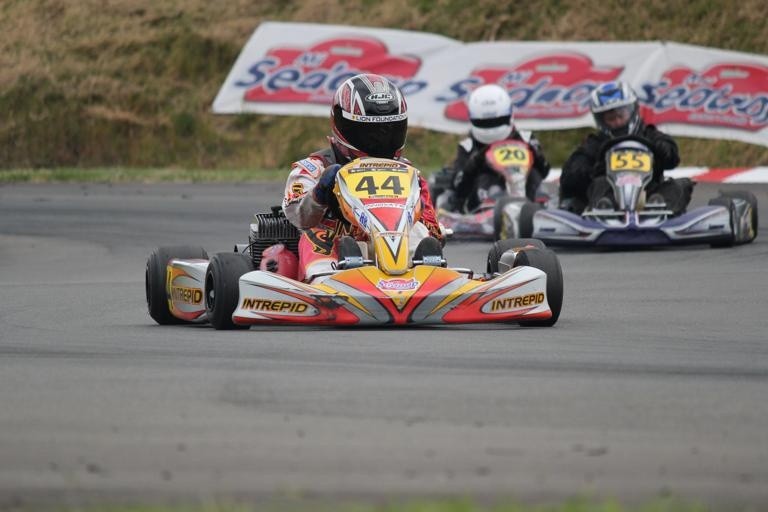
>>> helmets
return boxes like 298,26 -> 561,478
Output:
587,80 -> 645,141
328,73 -> 410,165
465,83 -> 516,145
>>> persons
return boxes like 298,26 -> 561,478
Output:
281,72 -> 445,284
559,80 -> 698,222
430,83 -> 551,213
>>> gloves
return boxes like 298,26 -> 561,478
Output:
311,164 -> 343,202
655,141 -> 673,165
